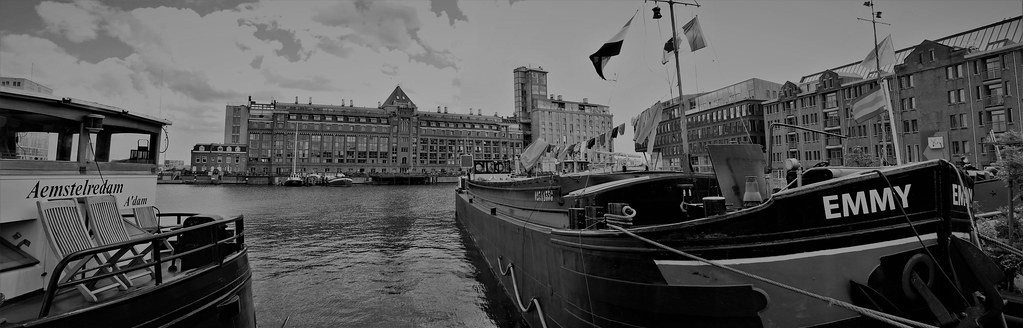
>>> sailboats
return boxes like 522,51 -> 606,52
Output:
284,123 -> 302,187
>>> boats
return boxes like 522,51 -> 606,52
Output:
305,169 -> 352,187
1,74 -> 258,328
456,0 -> 1009,328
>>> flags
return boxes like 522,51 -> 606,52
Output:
860,34 -> 898,70
589,10 -> 638,80
850,82 -> 889,124
682,15 -> 706,51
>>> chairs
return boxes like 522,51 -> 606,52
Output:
132,206 -> 182,254
83,195 -> 156,287
36,197 -> 128,303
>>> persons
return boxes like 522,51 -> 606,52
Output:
961,156 -> 980,171
